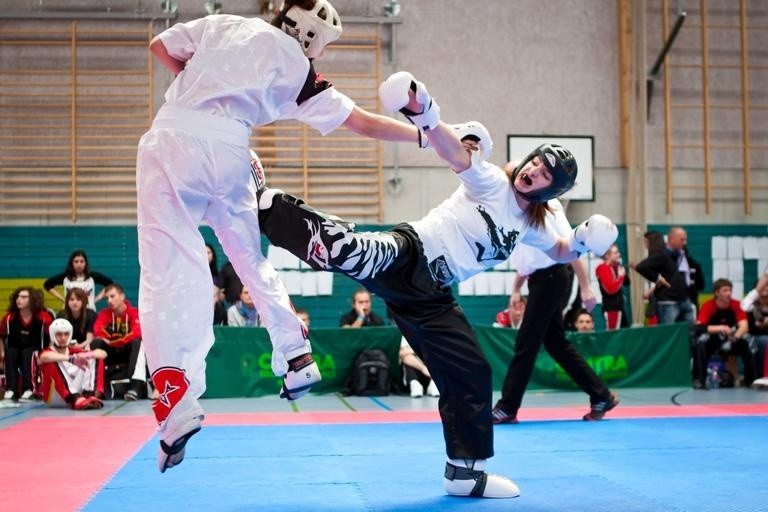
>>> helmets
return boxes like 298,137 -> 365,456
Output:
511,142 -> 578,203
50,318 -> 73,348
270,0 -> 343,59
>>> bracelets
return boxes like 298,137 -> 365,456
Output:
357,316 -> 365,321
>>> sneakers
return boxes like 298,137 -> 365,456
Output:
494,408 -> 520,424
126,391 -> 138,400
280,348 -> 321,402
75,396 -> 102,409
157,420 -> 201,472
5,390 -> 34,402
447,465 -> 520,498
249,151 -> 267,192
409,382 -> 439,397
694,379 -> 705,388
583,392 -> 618,420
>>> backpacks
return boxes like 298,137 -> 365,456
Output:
346,348 -> 393,396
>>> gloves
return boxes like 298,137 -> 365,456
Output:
571,214 -> 619,257
378,70 -> 441,130
418,121 -> 493,159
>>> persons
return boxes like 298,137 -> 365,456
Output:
496,244 -> 631,332
256,71 -> 619,499
630,227 -> 706,325
135,0 -> 493,473
205,244 -> 266,327
2,250 -> 147,411
492,160 -> 620,422
692,270 -> 768,390
398,335 -> 440,398
297,309 -> 311,327
339,288 -> 385,328
